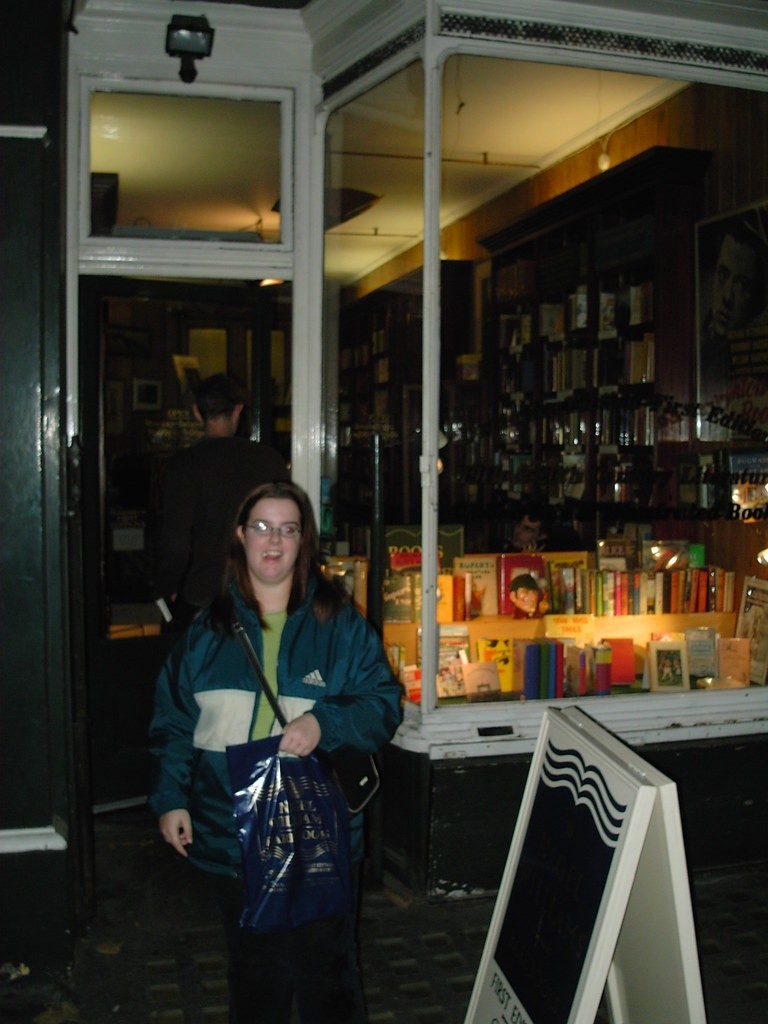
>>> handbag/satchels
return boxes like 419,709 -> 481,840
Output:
323,738 -> 381,815
226,733 -> 359,930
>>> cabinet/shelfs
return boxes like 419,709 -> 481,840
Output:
484,217 -> 694,516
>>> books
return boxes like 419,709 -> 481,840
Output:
324,539 -> 751,702
483,274 -> 655,538
335,330 -> 391,514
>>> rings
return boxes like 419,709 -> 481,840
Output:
533,546 -> 537,550
528,545 -> 532,549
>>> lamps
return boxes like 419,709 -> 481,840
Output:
166,14 -> 216,81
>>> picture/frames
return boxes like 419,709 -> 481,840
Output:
693,198 -> 768,442
132,379 -> 161,412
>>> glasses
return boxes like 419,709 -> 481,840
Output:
244,520 -> 301,539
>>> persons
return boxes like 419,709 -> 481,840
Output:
142,481 -> 408,1024
503,503 -> 553,552
143,371 -> 293,632
699,211 -> 768,388
